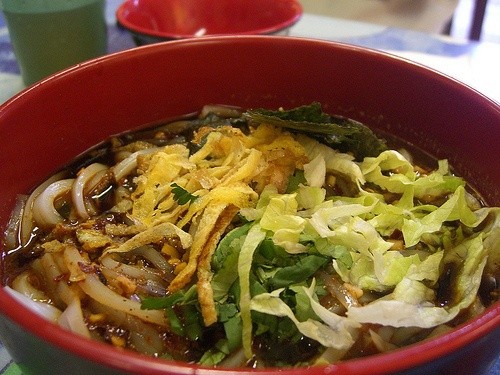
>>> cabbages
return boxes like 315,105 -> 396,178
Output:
138,134 -> 498,367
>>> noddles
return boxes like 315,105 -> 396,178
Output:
1,103 -> 500,369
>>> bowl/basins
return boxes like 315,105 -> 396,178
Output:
0,35 -> 499,375
116,0 -> 303,46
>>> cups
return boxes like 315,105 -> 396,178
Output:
0,0 -> 109,86
